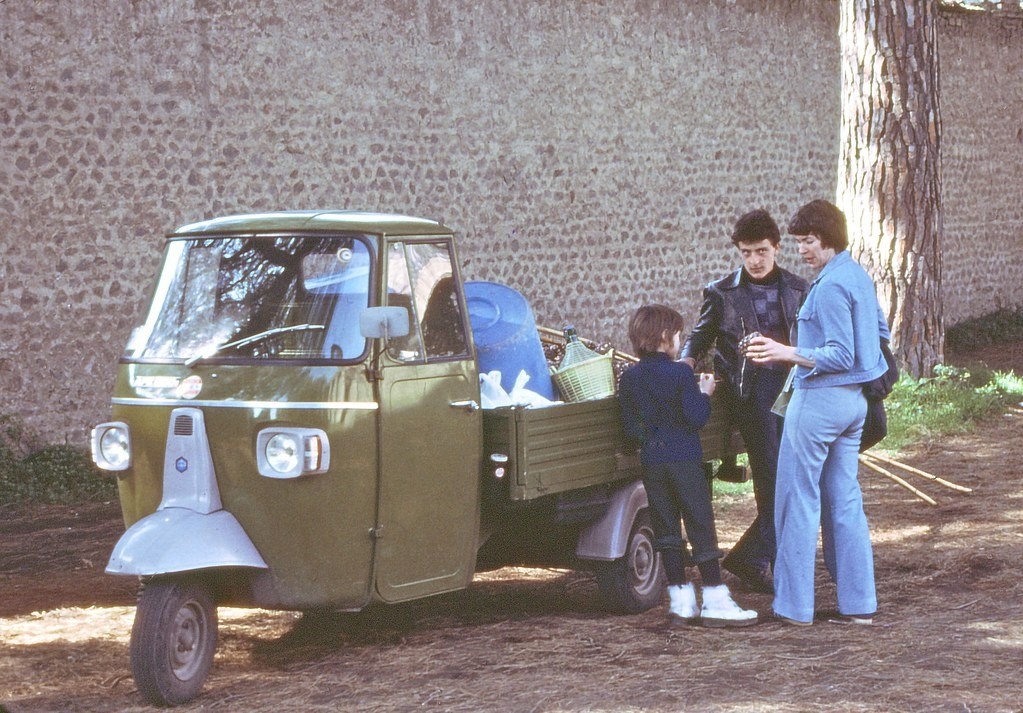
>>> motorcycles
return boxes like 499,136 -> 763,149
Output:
87,208 -> 754,709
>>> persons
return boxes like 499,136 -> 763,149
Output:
677,208 -> 813,594
746,198 -> 892,626
620,304 -> 759,626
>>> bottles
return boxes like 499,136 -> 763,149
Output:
558,325 -> 601,371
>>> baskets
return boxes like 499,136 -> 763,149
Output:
548,348 -> 615,400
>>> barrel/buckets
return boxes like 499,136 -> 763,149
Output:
463,282 -> 554,401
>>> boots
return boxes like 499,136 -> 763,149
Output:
699,585 -> 758,627
667,581 -> 700,620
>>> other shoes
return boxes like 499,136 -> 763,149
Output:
759,607 -> 813,626
814,608 -> 872,625
721,553 -> 774,595
